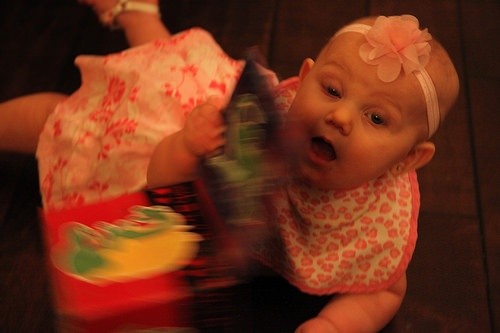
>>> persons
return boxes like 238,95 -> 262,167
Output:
0,0 -> 460,333
